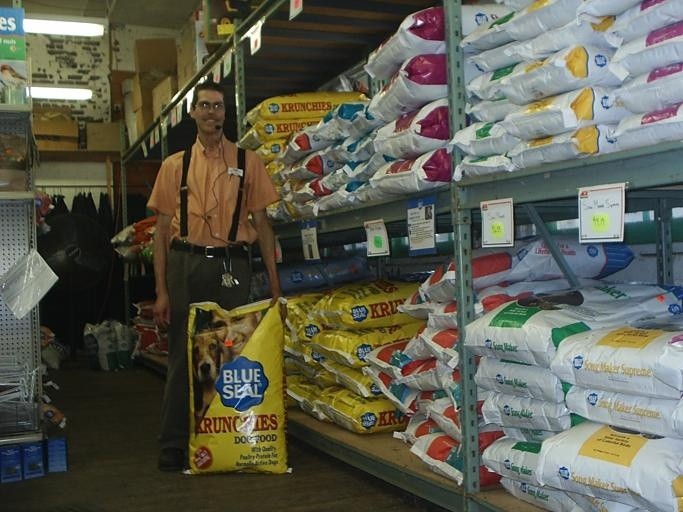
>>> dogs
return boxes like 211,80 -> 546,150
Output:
189,328 -> 232,426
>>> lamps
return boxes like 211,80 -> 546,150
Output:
23,12 -> 108,38
27,84 -> 94,102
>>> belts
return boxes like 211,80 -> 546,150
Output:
172,242 -> 241,258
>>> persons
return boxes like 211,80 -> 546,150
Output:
146,81 -> 287,472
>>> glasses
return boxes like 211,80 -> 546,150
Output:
195,101 -> 224,111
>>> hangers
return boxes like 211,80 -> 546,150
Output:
39,184 -> 114,202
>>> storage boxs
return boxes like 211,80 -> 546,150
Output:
121,11 -> 214,146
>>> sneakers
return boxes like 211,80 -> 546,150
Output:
159,447 -> 185,471
